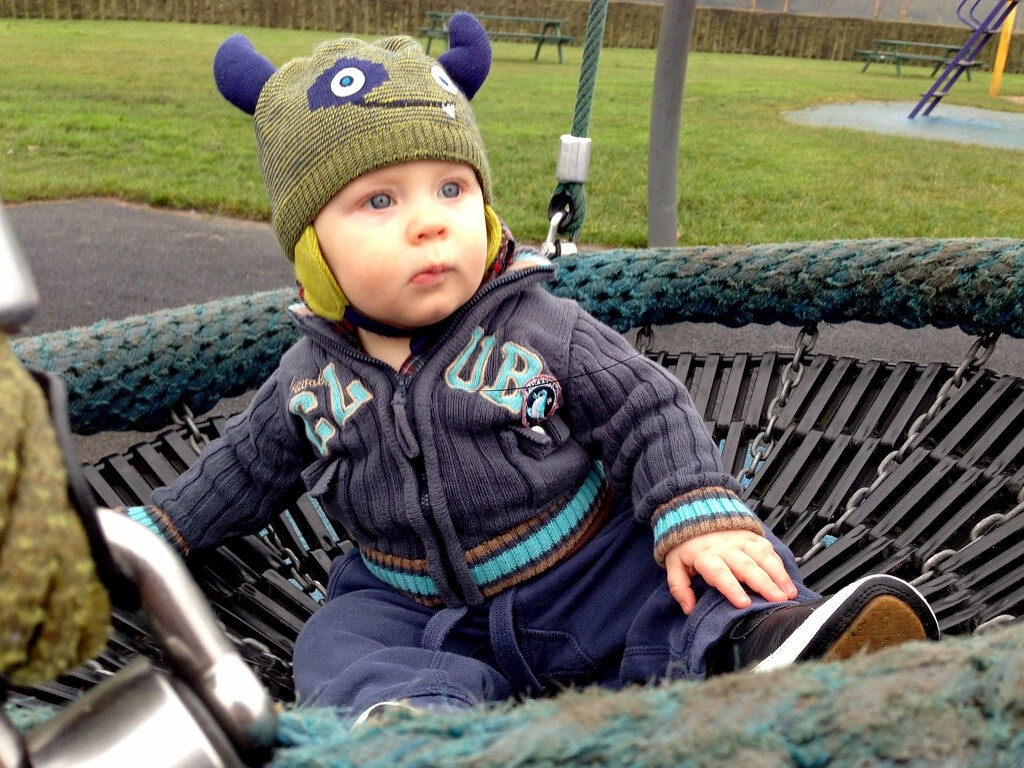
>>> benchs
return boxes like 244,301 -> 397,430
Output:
854,50 -> 946,63
422,11 -> 571,64
898,53 -> 982,67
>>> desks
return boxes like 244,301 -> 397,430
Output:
861,39 -> 983,81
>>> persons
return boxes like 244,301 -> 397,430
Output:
118,14 -> 943,734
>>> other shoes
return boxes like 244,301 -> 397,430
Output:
722,573 -> 939,679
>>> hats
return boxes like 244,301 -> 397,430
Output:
215,12 -> 502,339
